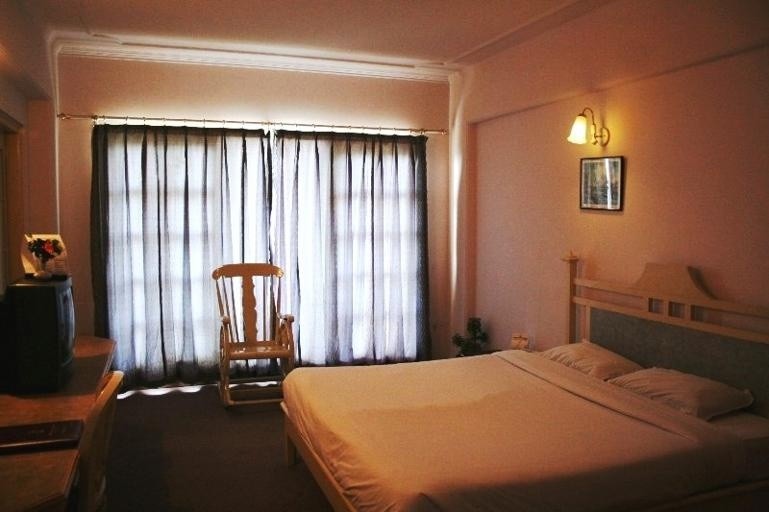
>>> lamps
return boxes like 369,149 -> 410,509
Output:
564,108 -> 610,147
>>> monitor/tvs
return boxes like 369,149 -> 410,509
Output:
0,278 -> 76,394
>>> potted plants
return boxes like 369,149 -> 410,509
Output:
452,317 -> 489,356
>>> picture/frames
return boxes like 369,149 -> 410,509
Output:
578,155 -> 625,212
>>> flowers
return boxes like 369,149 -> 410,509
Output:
27,238 -> 64,263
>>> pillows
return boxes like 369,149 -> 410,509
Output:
540,340 -> 754,420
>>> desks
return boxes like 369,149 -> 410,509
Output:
0,333 -> 116,512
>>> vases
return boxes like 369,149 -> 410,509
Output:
33,260 -> 52,280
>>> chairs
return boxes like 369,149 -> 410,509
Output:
212,263 -> 295,410
78,370 -> 125,512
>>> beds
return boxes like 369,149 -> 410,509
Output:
280,249 -> 768,509
